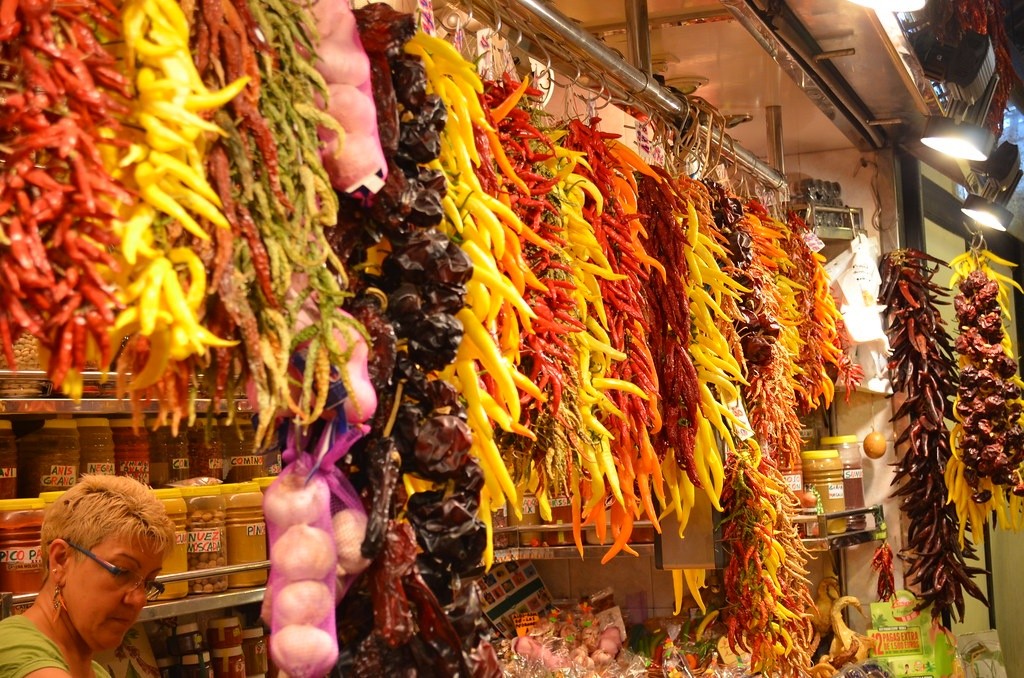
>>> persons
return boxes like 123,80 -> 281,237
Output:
1,473 -> 179,677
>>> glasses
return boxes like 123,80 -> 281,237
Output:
47,539 -> 165,602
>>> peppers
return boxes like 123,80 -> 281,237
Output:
1,0 -> 1024,678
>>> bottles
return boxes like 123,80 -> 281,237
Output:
2,325 -> 296,678
801,449 -> 849,534
799,483 -> 819,539
778,450 -> 805,538
795,410 -> 815,455
821,435 -> 867,532
